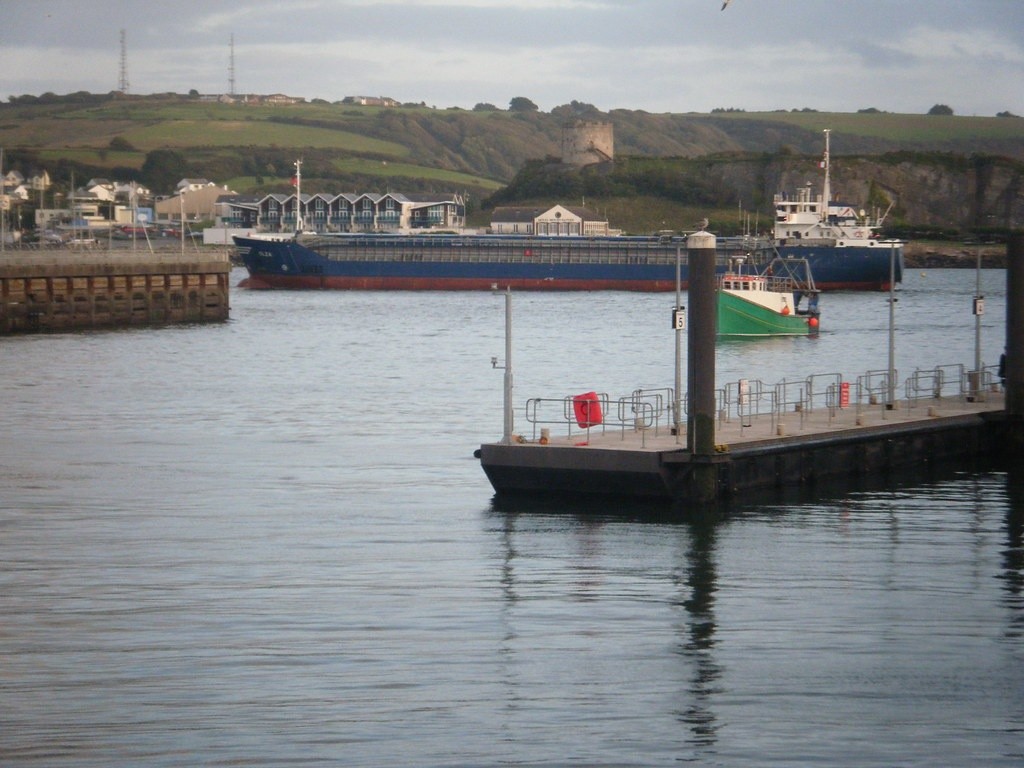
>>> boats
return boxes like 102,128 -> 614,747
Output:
231,123 -> 910,292
712,251 -> 822,335
